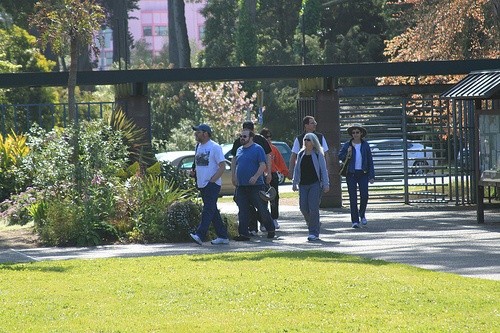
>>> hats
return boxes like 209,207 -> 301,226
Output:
192,124 -> 212,134
347,123 -> 368,137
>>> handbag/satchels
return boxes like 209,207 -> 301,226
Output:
339,145 -> 352,176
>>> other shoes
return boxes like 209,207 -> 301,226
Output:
248,230 -> 257,235
268,231 -> 275,238
236,235 -> 250,240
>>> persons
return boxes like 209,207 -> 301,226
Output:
260,127 -> 292,233
338,124 -> 375,228
231,121 -> 272,236
234,131 -> 275,242
293,133 -> 329,240
289,116 -> 329,226
190,123 -> 229,245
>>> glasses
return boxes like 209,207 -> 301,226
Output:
266,136 -> 270,138
304,138 -> 311,141
240,135 -> 249,138
351,131 -> 360,134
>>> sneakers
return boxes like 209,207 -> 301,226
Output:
361,217 -> 367,224
190,233 -> 203,245
211,237 -> 230,244
260,223 -> 267,232
352,222 -> 359,228
307,235 -> 319,241
273,219 -> 280,229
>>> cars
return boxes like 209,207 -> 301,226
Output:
155,151 -> 236,196
219,141 -> 293,185
367,139 -> 439,175
